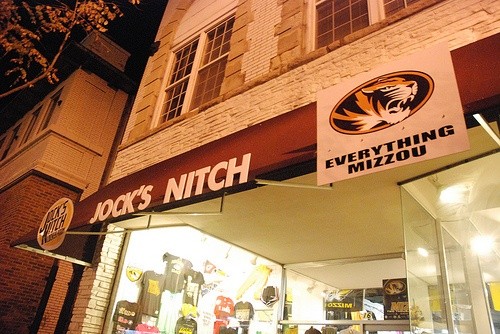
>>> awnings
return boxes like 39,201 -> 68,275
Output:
10,32 -> 499,269
